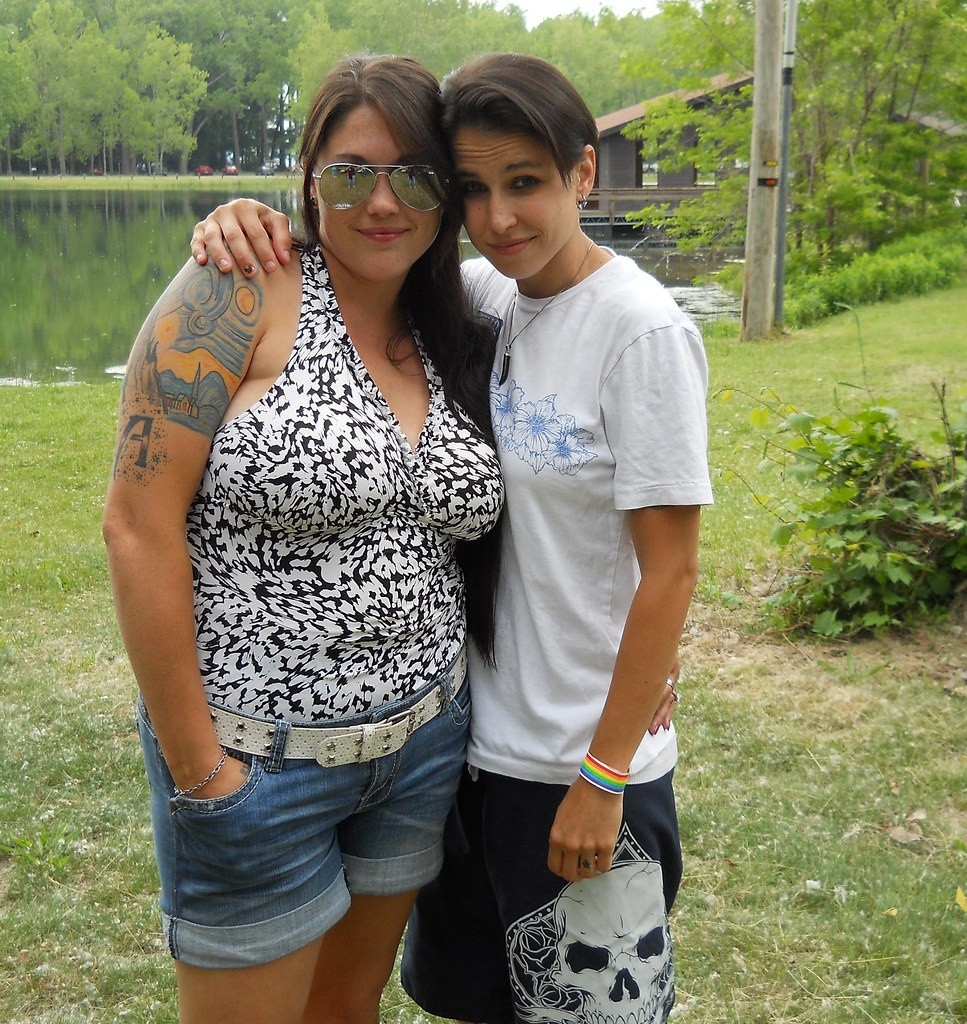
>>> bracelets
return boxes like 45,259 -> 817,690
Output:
580,753 -> 630,795
173,746 -> 229,795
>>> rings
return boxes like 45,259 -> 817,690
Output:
667,679 -> 675,691
673,691 -> 681,703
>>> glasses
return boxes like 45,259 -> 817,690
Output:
305,162 -> 450,211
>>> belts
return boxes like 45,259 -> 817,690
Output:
206,644 -> 471,769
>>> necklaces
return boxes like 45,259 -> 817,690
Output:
500,240 -> 593,387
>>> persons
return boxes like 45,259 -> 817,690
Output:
105,54 -> 681,1024
346,165 -> 355,188
406,168 -> 416,189
189,53 -> 714,1024
